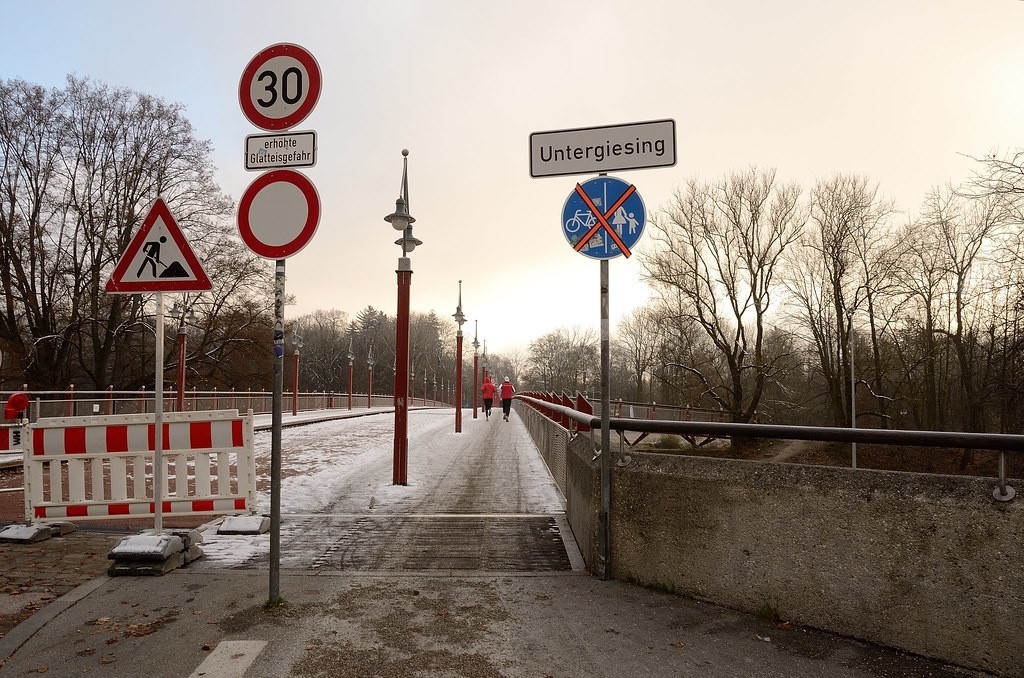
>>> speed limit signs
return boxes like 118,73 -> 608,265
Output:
238,42 -> 324,131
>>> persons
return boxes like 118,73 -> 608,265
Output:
499,376 -> 516,422
481,377 -> 495,421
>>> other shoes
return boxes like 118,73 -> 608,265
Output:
506,417 -> 508,422
486,418 -> 489,421
503,414 -> 506,420
489,410 -> 491,416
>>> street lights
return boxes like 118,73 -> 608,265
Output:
471,319 -> 480,418
424,369 -> 427,406
411,362 -> 415,406
366,345 -> 374,408
441,377 -> 443,407
292,334 -> 304,416
481,339 -> 487,412
433,373 -> 437,407
172,293 -> 198,412
393,355 -> 396,406
452,280 -> 467,433
447,380 -> 450,407
347,337 -> 355,411
383,149 -> 423,486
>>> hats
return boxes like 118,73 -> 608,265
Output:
504,377 -> 509,382
